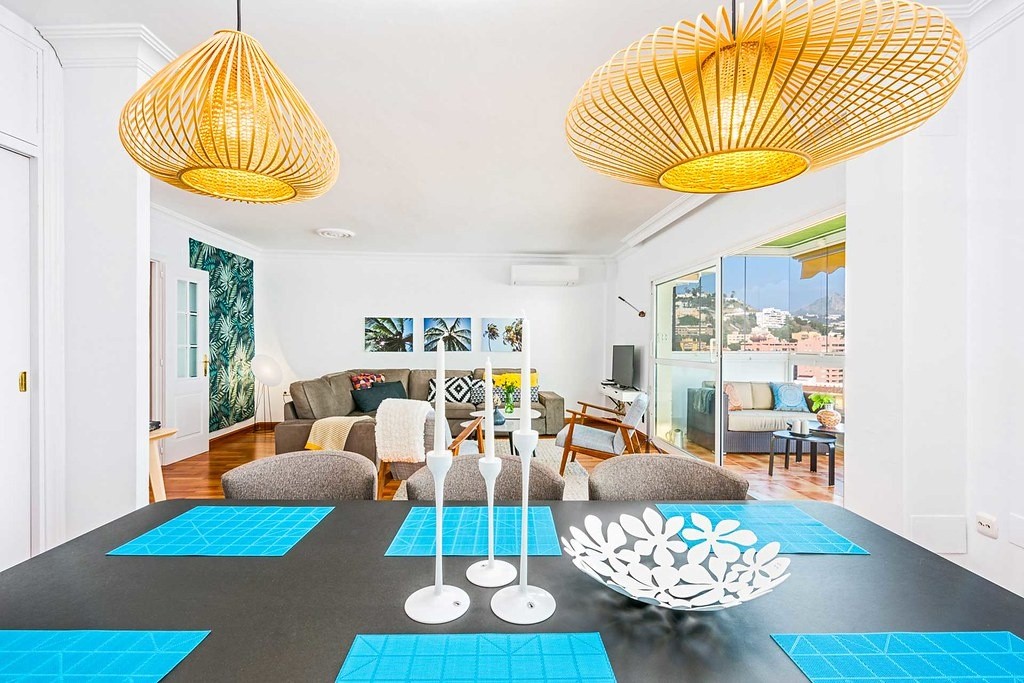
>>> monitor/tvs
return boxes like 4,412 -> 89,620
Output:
612,345 -> 634,388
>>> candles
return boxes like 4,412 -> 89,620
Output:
520,310 -> 532,432
433,336 -> 445,452
485,355 -> 495,456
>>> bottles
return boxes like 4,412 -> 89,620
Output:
493,405 -> 505,426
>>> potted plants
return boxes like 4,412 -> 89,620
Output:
807,390 -> 842,427
503,375 -> 518,413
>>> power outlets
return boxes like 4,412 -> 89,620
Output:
282,389 -> 290,396
976,511 -> 998,540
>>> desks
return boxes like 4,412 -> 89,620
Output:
459,418 -> 538,457
600,381 -> 645,424
149,426 -> 180,503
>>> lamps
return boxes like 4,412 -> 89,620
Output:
562,0 -> 974,195
619,295 -> 646,317
250,351 -> 283,434
112,0 -> 341,208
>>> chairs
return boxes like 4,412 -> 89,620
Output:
404,454 -> 563,501
552,390 -> 651,478
219,451 -> 380,500
590,452 -> 750,500
373,397 -> 487,501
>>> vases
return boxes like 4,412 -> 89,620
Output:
494,405 -> 506,425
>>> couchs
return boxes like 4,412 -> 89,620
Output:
275,367 -> 565,466
687,380 -> 840,456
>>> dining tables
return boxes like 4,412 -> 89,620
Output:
0,503 -> 1024,683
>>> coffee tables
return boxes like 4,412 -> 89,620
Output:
784,418 -> 844,473
469,407 -> 545,456
768,429 -> 838,487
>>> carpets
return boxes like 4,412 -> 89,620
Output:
391,438 -> 591,502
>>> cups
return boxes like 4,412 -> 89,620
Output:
802,420 -> 809,434
793,420 -> 801,434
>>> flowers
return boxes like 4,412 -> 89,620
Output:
494,393 -> 503,406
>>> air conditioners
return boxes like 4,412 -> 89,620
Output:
509,264 -> 580,287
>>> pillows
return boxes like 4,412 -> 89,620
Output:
503,386 -> 521,402
769,382 -> 810,413
493,387 -> 503,402
470,377 -> 493,403
713,381 -> 744,411
482,371 -> 539,389
426,373 -> 473,402
360,372 -> 386,384
349,387 -> 386,411
531,384 -> 542,403
350,374 -> 376,389
372,380 -> 407,399
